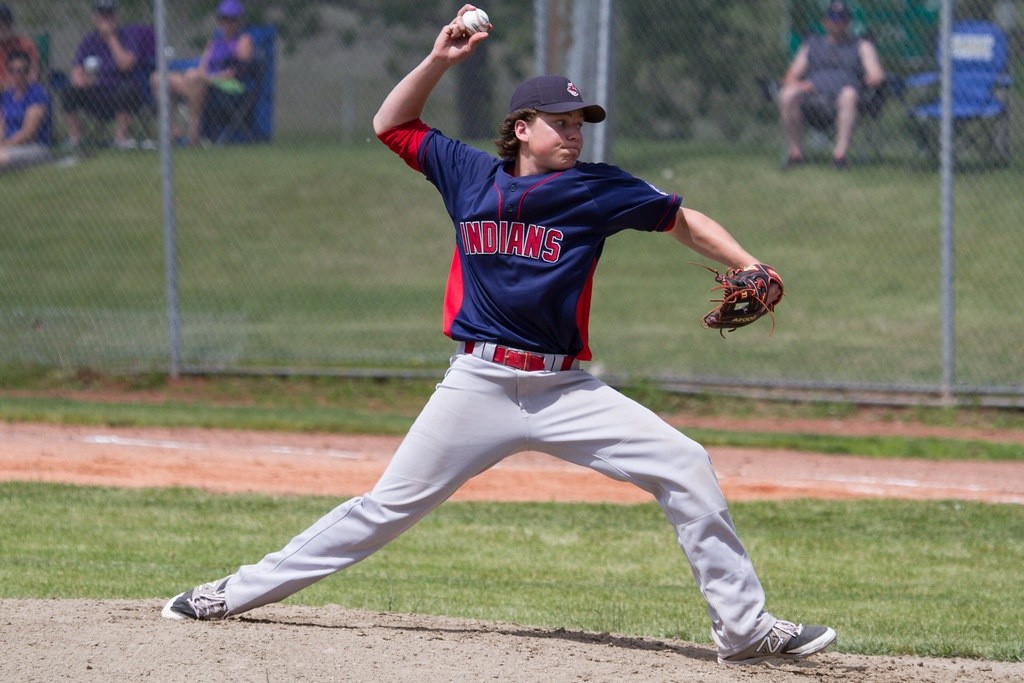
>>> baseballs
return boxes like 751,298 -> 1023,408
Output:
462,7 -> 490,37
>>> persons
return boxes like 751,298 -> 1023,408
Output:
0,0 -> 52,166
913,1 -> 1010,168
774,0 -> 885,171
49,0 -> 155,155
161,3 -> 836,664
149,0 -> 274,149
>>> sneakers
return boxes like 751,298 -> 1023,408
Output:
161,573 -> 232,621
717,620 -> 837,666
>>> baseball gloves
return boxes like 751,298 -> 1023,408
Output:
686,259 -> 785,340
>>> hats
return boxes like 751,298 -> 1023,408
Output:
820,0 -> 851,23
93,1 -> 116,10
218,0 -> 244,18
510,76 -> 607,123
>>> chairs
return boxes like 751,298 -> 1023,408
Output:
905,21 -> 1013,169
31,24 -> 275,154
754,25 -> 896,164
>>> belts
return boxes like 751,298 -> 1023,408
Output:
463,339 -> 573,372
